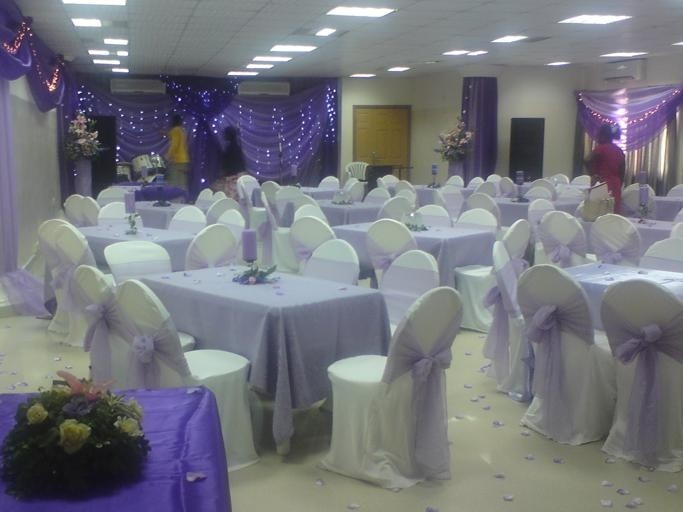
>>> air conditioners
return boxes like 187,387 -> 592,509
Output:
237,80 -> 292,97
109,78 -> 168,96
604,58 -> 648,83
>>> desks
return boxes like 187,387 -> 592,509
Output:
2,384 -> 224,512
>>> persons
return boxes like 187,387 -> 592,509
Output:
586,123 -> 627,213
163,115 -> 190,191
213,126 -> 253,197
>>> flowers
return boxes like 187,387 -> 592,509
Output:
1,369 -> 149,503
433,117 -> 474,163
64,112 -> 101,159
